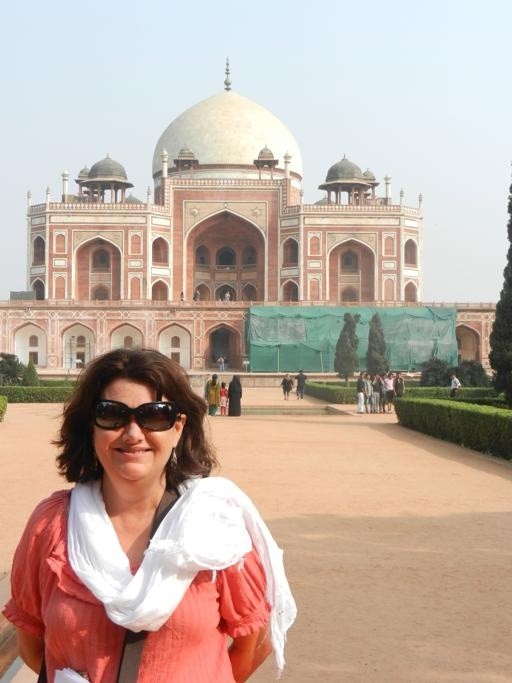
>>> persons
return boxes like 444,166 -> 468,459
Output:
225,290 -> 231,301
193,287 -> 201,301
295,369 -> 307,400
216,355 -> 224,371
205,373 -> 221,416
357,370 -> 405,413
281,373 -> 294,399
180,291 -> 185,301
450,373 -> 460,397
228,374 -> 242,415
219,381 -> 229,415
0,346 -> 299,683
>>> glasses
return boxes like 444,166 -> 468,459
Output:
91,398 -> 181,432
